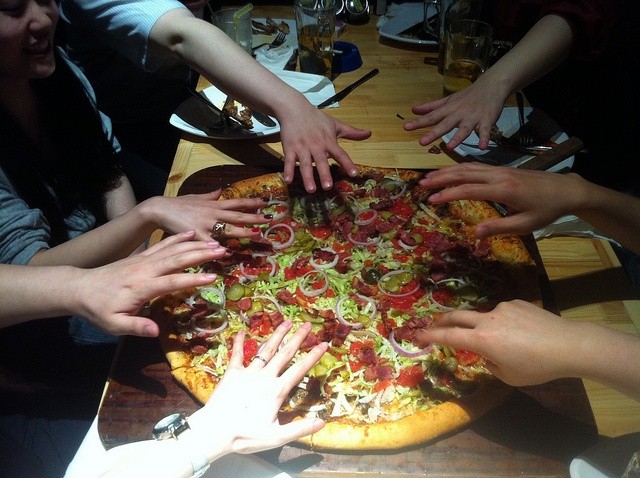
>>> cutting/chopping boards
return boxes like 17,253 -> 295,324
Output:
97,164 -> 600,474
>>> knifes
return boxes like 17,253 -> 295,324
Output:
315,66 -> 380,112
246,106 -> 277,128
479,143 -> 555,155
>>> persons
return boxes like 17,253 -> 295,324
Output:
412,299 -> 639,402
0,231 -> 226,471
0,0 -> 273,369
403,1 -> 640,151
424,163 -> 640,256
81,318 -> 330,478
55,0 -> 373,203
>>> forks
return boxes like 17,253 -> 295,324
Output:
252,31 -> 291,52
513,90 -> 533,150
187,84 -> 250,136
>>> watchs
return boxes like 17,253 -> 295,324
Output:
152,412 -> 211,478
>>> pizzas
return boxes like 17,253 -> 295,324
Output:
149,158 -> 543,452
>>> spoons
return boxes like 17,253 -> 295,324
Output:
205,93 -> 236,133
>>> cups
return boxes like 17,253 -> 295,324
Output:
443,19 -> 514,93
423,0 -> 487,72
294,0 -> 336,75
211,9 -> 252,60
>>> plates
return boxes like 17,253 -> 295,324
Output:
438,106 -> 576,174
250,17 -> 343,66
168,83 -> 281,140
376,2 -> 444,46
569,431 -> 640,478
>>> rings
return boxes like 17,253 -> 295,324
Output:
213,220 -> 225,234
251,354 -> 268,366
